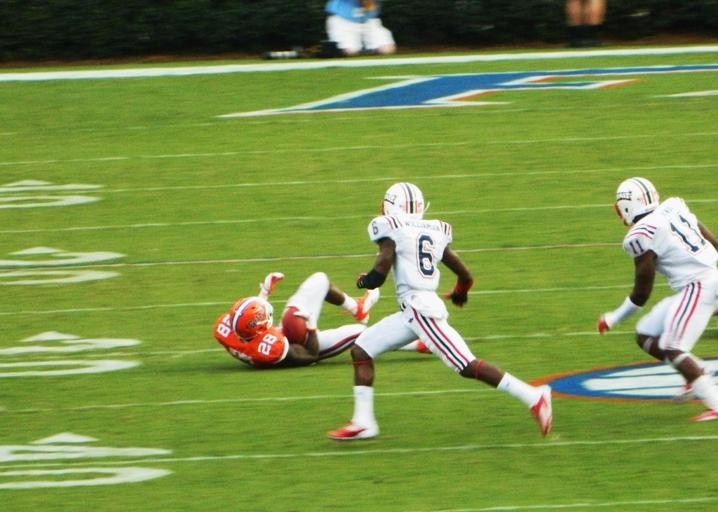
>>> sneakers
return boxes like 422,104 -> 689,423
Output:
526,384 -> 554,439
352,288 -> 380,325
672,382 -> 718,425
327,419 -> 381,441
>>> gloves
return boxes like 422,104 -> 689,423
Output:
596,311 -> 616,334
258,271 -> 284,295
442,291 -> 469,309
355,273 -> 367,288
285,303 -> 318,332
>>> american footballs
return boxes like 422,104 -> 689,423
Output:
282,306 -> 308,346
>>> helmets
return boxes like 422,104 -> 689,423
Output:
229,296 -> 273,342
612,176 -> 662,228
381,181 -> 426,217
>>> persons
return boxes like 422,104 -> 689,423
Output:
557,0 -> 605,48
597,177 -> 718,424
213,272 -> 432,368
324,0 -> 396,57
329,181 -> 551,440
